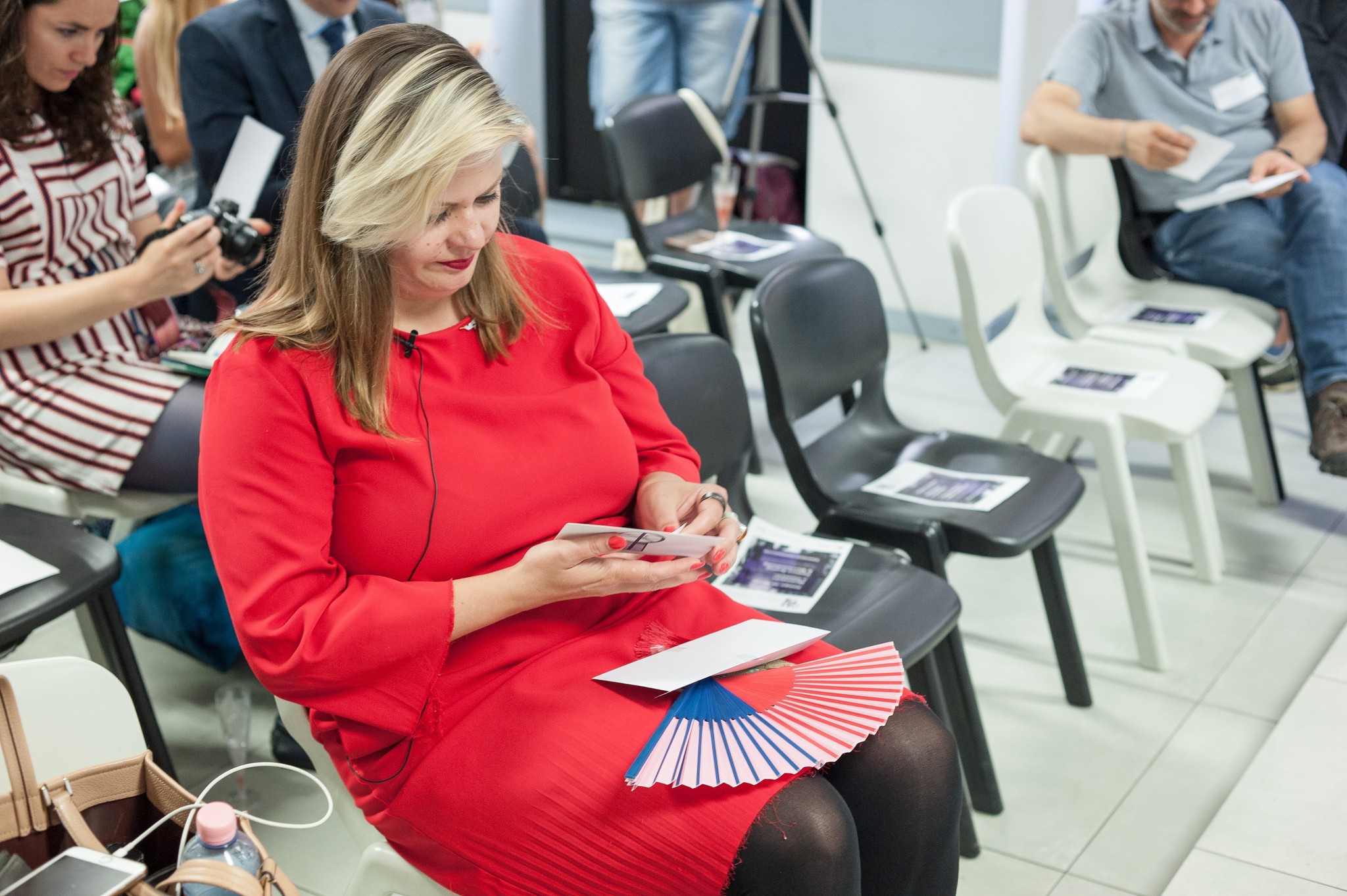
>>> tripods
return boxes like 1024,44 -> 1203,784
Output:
726,0 -> 944,355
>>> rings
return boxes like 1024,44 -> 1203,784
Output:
698,492 -> 726,514
196,261 -> 207,274
720,511 -> 747,542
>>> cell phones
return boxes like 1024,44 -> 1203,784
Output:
2,845 -> 150,895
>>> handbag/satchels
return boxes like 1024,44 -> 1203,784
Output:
0,674 -> 298,896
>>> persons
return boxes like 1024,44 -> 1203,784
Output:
1020,0 -> 1347,479
176,0 -> 549,308
134,0 -> 237,220
587,0 -> 758,273
195,23 -> 963,896
0,0 -> 320,771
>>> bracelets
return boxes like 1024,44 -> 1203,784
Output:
1271,146 -> 1293,159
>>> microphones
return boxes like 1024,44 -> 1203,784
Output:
405,330 -> 418,358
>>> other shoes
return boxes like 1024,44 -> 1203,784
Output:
272,714 -> 316,774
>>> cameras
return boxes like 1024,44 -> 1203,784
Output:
174,199 -> 258,257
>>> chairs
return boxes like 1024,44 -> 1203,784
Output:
0,88 -> 1291,896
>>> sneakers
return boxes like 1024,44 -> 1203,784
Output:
1309,381 -> 1347,477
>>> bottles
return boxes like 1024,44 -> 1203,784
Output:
180,801 -> 262,896
